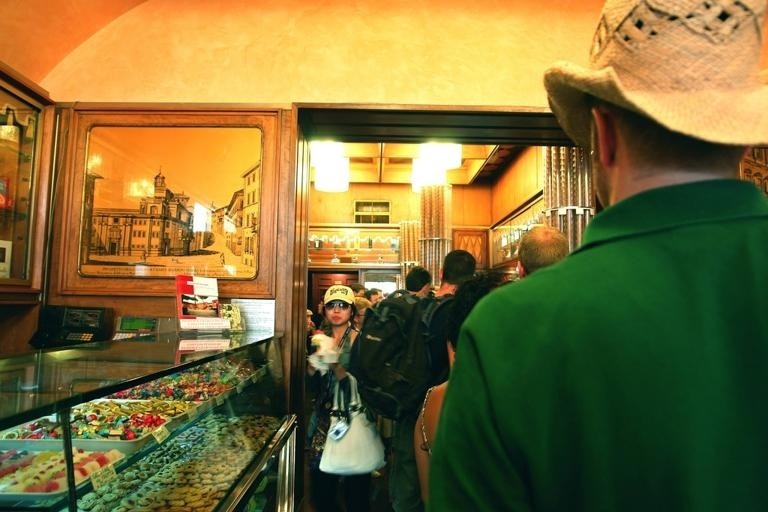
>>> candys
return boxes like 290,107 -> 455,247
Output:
3,355 -> 254,440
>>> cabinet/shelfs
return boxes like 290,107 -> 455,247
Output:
353,199 -> 390,224
0,328 -> 299,512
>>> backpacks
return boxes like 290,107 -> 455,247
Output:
357,290 -> 454,423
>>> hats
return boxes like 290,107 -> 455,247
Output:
324,285 -> 355,307
544,1 -> 768,147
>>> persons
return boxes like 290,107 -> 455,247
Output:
306,224 -> 570,511
424,0 -> 768,512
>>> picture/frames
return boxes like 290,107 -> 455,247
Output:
0,59 -> 58,307
56,106 -> 283,300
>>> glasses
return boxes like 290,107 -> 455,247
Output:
326,302 -> 349,310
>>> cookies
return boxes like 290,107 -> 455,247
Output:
0,415 -> 281,512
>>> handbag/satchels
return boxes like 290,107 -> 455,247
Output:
320,373 -> 386,476
